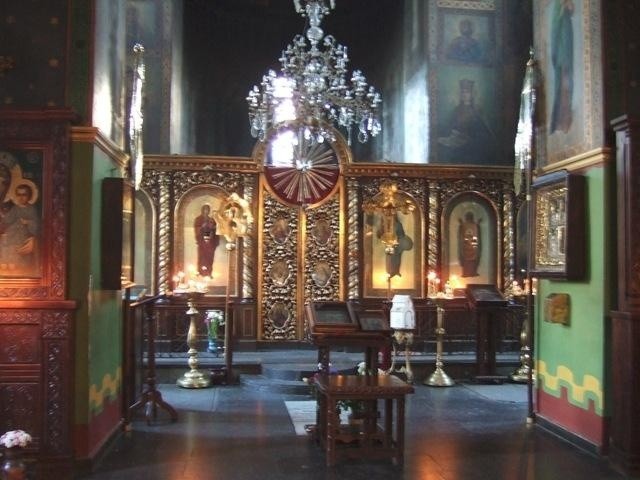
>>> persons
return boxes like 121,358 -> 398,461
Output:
447,79 -> 492,147
457,212 -> 483,276
194,205 -> 220,279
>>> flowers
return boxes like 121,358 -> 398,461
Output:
0,429 -> 35,450
204,310 -> 225,338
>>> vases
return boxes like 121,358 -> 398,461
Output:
207,338 -> 217,352
0,445 -> 29,480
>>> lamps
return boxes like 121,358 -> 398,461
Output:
420,272 -> 460,388
240,1 -> 383,153
172,264 -> 214,389
510,278 -> 532,384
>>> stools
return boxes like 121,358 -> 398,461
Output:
311,372 -> 416,470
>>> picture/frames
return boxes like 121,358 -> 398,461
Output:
0,117 -> 71,302
